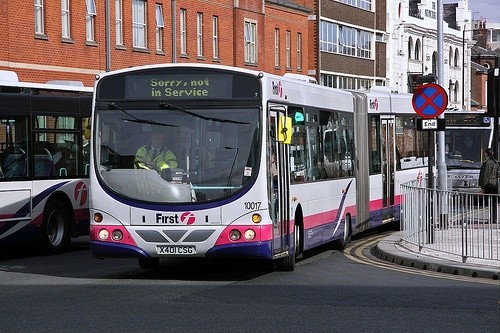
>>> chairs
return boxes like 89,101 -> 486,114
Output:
0,143 -> 89,181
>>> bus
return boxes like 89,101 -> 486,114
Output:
88,61 -> 438,275
440,108 -> 500,204
0,70 -> 95,254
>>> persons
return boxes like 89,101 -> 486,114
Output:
479,148 -> 499,224
134,129 -> 179,176
